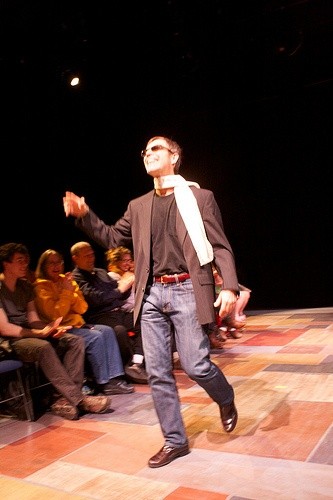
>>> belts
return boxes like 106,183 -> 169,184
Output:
150,272 -> 191,284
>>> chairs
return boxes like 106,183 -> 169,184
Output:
0,360 -> 50,422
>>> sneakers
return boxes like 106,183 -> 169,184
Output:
81,395 -> 112,413
51,397 -> 80,420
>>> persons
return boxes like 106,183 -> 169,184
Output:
0,242 -> 251,420
63,136 -> 240,468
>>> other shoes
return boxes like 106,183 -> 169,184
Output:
209,330 -> 224,349
230,328 -> 241,339
125,363 -> 149,384
104,379 -> 134,395
217,329 -> 227,341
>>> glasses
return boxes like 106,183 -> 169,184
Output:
46,260 -> 64,267
141,145 -> 174,157
119,259 -> 132,263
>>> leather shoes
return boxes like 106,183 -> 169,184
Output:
220,399 -> 239,433
148,444 -> 190,468
